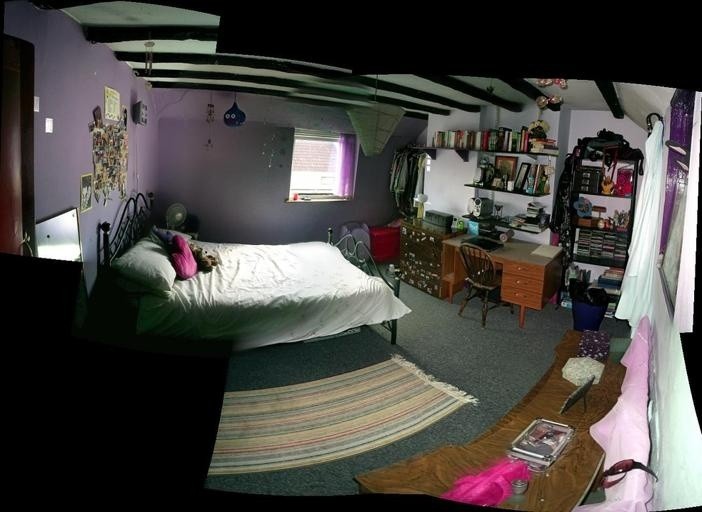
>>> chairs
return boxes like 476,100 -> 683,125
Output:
457,243 -> 513,327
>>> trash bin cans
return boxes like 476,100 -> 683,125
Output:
572,288 -> 610,332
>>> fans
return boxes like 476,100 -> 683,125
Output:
165,202 -> 187,230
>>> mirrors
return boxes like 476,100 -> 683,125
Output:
33,207 -> 90,332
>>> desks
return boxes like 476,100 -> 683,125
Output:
352,330 -> 632,512
442,233 -> 562,328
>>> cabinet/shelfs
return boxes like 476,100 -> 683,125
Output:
398,219 -> 467,299
554,155 -> 640,311
461,149 -> 558,235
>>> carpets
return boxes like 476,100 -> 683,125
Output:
206,354 -> 479,478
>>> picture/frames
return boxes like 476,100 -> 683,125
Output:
510,417 -> 575,467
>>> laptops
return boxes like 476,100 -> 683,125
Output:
461,237 -> 504,252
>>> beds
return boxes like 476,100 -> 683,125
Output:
99,191 -> 403,346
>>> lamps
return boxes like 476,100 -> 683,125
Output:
344,75 -> 407,158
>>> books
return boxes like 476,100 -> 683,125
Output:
433,127 -> 559,155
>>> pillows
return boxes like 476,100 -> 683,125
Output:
110,225 -> 197,300
